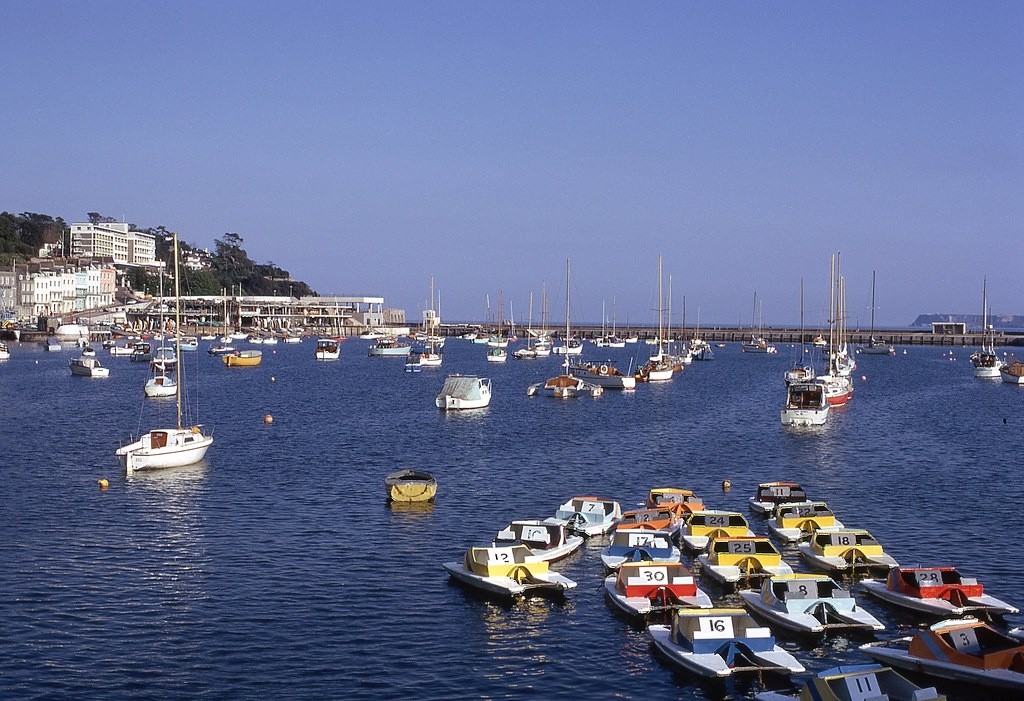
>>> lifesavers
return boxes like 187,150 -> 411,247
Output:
601,365 -> 608,373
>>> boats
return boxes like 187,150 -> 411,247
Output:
130,342 -> 151,364
780,383 -> 831,427
42,337 -> 61,352
562,360 -> 636,389
0,341 -> 10,360
441,482 -> 1024,701
150,346 -> 177,370
68,357 -> 109,378
435,373 -> 492,409
385,469 -> 437,502
222,349 -> 262,365
998,352 -> 1024,384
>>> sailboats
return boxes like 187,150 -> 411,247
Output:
114,233 -> 213,474
527,257 -> 603,399
144,258 -> 177,397
207,288 -> 236,356
54,253 -> 895,407
969,276 -> 1007,376
